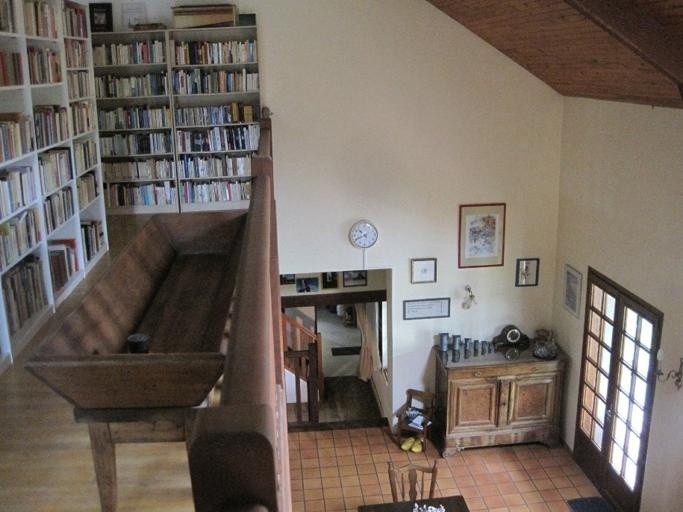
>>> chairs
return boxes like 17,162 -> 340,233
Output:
386,459 -> 437,502
393,388 -> 437,452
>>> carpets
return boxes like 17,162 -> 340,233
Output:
322,374 -> 380,424
566,495 -> 613,511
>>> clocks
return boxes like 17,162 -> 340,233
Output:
493,324 -> 529,350
350,219 -> 379,249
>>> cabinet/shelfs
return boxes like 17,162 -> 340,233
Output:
167,21 -> 263,215
433,339 -> 569,458
89,29 -> 179,215
0,1 -> 111,374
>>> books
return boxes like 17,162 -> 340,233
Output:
0,1 -> 107,335
407,415 -> 432,431
90,40 -> 257,209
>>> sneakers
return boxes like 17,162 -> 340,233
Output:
400,436 -> 415,451
410,438 -> 422,453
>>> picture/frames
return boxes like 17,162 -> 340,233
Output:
278,274 -> 297,287
321,272 -> 337,289
410,259 -> 437,285
514,257 -> 539,287
295,276 -> 320,294
343,270 -> 366,287
458,202 -> 505,268
402,297 -> 450,320
560,263 -> 583,319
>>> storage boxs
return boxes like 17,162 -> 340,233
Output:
171,2 -> 240,29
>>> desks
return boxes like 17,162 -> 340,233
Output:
358,494 -> 470,512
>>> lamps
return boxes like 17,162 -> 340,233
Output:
652,348 -> 682,389
463,284 -> 476,309
